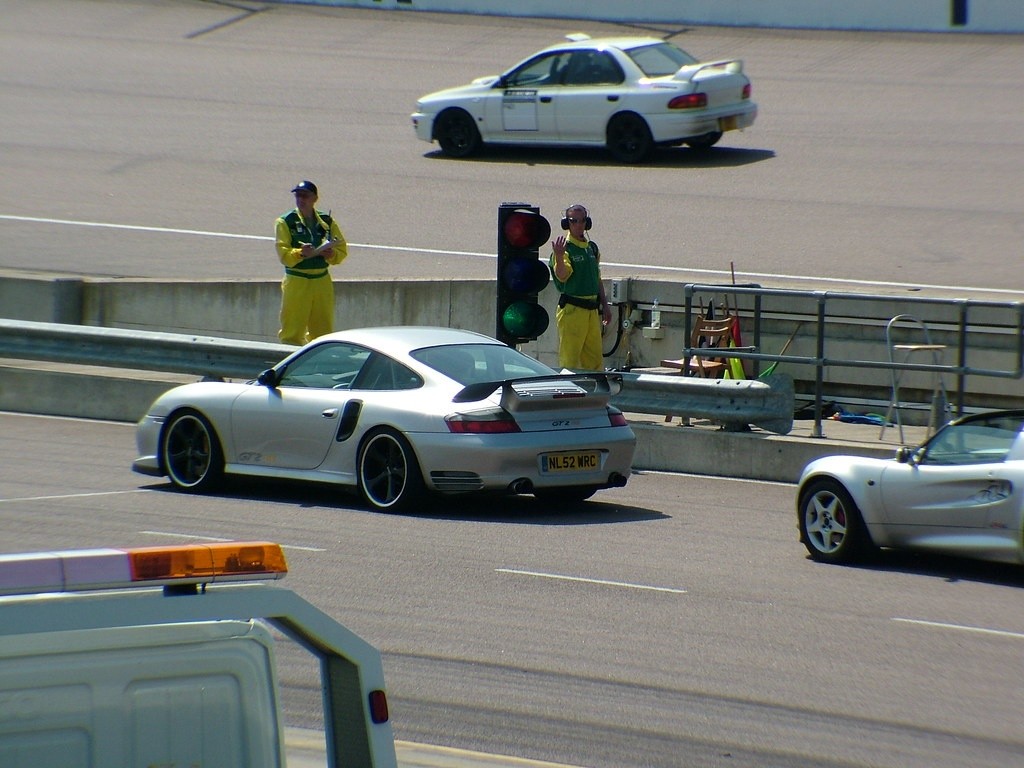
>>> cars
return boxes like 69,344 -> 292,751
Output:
408,32 -> 760,163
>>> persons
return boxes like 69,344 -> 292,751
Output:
548,205 -> 612,372
275,180 -> 348,347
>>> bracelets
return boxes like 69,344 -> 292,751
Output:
300,254 -> 307,257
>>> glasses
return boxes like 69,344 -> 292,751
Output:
293,192 -> 315,199
568,217 -> 587,224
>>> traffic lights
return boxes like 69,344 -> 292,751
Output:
498,201 -> 552,346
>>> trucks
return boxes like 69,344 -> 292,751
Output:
0,536 -> 397,768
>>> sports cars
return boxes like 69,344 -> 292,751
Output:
130,324 -> 639,514
793,408 -> 1024,565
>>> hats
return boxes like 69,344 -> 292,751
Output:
290,180 -> 317,195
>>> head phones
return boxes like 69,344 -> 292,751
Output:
561,205 -> 592,230
